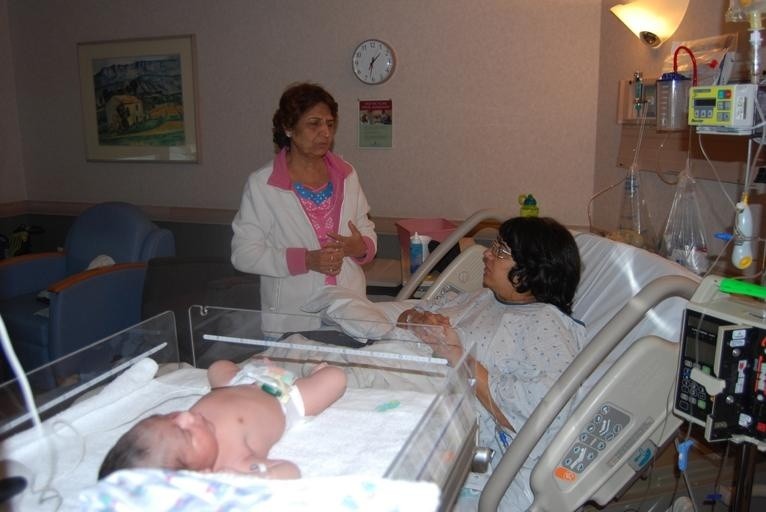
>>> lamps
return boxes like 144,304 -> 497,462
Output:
610,0 -> 690,50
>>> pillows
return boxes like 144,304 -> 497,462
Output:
297,285 -> 426,344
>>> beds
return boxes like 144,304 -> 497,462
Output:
393,208 -> 731,512
1,304 -> 496,512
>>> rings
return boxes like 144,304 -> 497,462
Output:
329,268 -> 333,275
330,254 -> 335,261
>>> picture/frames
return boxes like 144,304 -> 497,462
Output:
76,34 -> 200,163
357,98 -> 395,151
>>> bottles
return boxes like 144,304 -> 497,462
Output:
518,192 -> 538,219
655,72 -> 691,134
410,232 -> 423,274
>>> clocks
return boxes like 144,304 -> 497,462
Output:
351,39 -> 397,85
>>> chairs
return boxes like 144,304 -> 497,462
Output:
0,202 -> 162,390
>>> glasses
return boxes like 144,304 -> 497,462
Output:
488,240 -> 512,259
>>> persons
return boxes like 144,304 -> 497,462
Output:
228,80 -> 379,352
390,217 -> 588,511
98,355 -> 350,484
